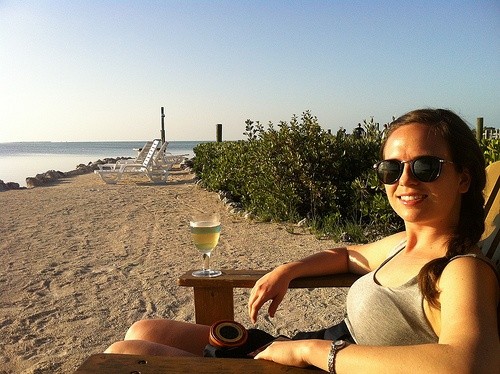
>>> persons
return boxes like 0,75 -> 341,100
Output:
104,108 -> 500,374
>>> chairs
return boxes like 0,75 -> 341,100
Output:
73,161 -> 500,374
93,138 -> 189,184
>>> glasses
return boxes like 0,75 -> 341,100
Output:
376,155 -> 458,185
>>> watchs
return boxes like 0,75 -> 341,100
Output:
328,339 -> 350,374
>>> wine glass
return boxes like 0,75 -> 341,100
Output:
189,217 -> 223,278
